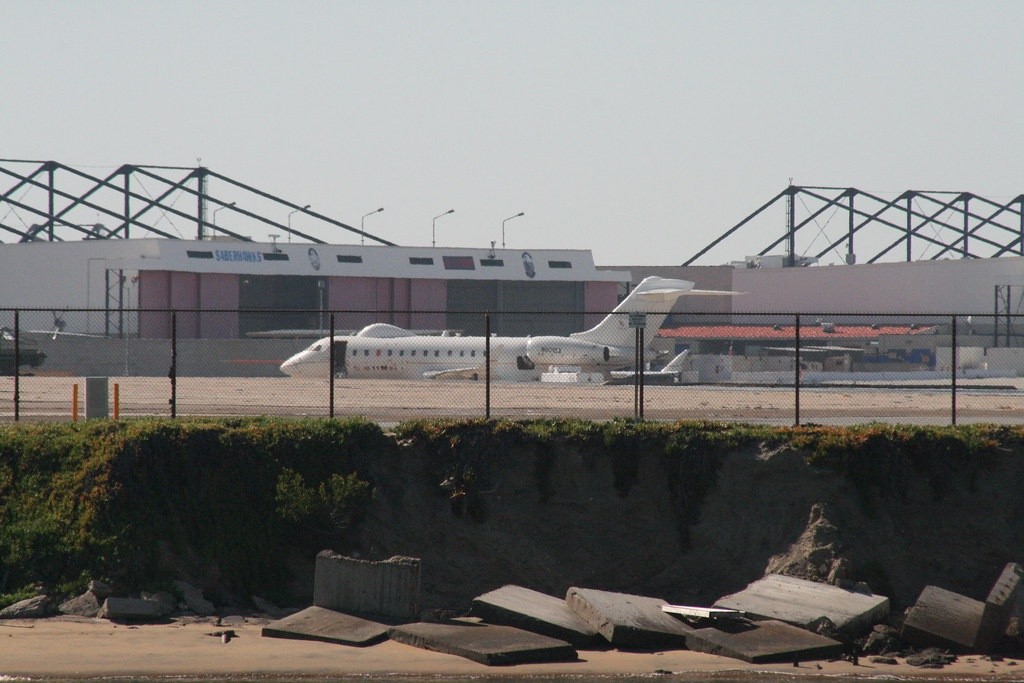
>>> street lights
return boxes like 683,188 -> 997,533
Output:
432,209 -> 456,247
361,207 -> 384,246
213,201 -> 237,240
502,213 -> 524,249
288,205 -> 311,244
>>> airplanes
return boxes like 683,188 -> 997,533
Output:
279,275 -> 749,387
0,305 -> 117,376
